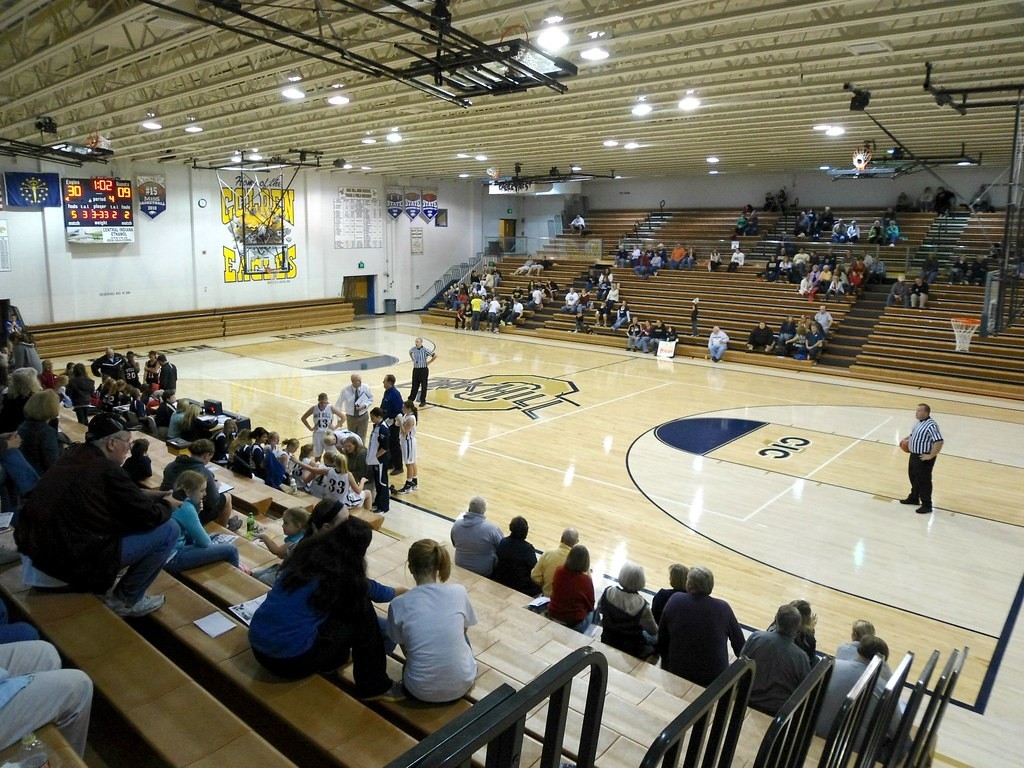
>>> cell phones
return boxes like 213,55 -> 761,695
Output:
172,487 -> 188,501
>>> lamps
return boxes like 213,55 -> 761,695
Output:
143,9 -> 972,176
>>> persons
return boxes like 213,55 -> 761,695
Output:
442,254 -> 559,333
735,184 -> 996,247
690,297 -> 701,337
569,214 -> 585,235
0,314 -> 418,615
899,403 -> 943,515
613,243 -> 991,310
451,497 -> 916,768
560,267 -> 834,367
405,338 -> 438,408
706,325 -> 729,363
246,498 -> 477,706
0,621 -> 94,767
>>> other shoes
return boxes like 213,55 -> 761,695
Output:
419,402 -> 426,406
390,468 -> 404,475
228,516 -> 243,532
373,508 -> 385,514
900,498 -> 920,505
916,506 -> 932,513
595,323 -> 600,326
633,348 -> 636,352
813,361 -> 817,366
404,399 -> 412,403
626,348 -> 631,351
604,323 -> 606,327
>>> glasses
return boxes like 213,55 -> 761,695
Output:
113,436 -> 135,449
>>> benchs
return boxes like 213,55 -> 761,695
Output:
0,404 -> 942,768
422,204 -> 1024,400
29,294 -> 355,359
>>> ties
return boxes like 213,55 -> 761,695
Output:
353,388 -> 359,418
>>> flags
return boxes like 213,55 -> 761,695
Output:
2,171 -> 62,207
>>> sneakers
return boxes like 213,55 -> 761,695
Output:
412,482 -> 418,490
106,594 -> 165,618
365,680 -> 405,702
397,485 -> 412,494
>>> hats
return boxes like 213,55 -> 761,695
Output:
84,413 -> 143,442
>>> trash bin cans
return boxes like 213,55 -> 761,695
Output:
385,299 -> 396,316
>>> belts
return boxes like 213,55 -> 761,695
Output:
348,411 -> 366,418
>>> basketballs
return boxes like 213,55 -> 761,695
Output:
901,440 -> 911,453
